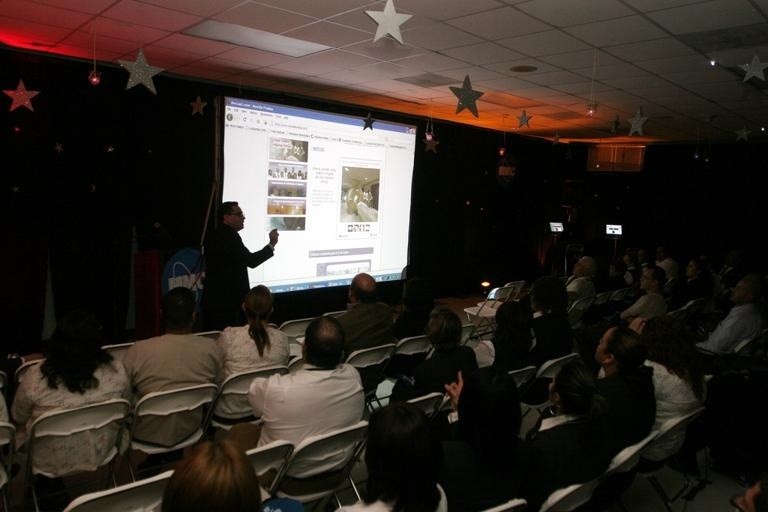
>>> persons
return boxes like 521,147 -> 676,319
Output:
202,285 -> 290,441
603,249 -> 764,380
391,276 -> 608,510
640,340 -> 706,460
565,255 -> 598,316
335,404 -> 449,510
246,316 -> 367,484
10,312 -> 134,509
161,438 -> 262,509
336,273 -> 393,363
593,324 -> 656,503
198,200 -> 280,327
122,287 -> 222,478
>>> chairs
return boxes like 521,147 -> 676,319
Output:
1,272 -> 768,510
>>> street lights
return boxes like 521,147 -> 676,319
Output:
168,261 -> 205,305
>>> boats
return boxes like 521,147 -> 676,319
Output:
614,144 -> 647,173
587,144 -> 613,171
